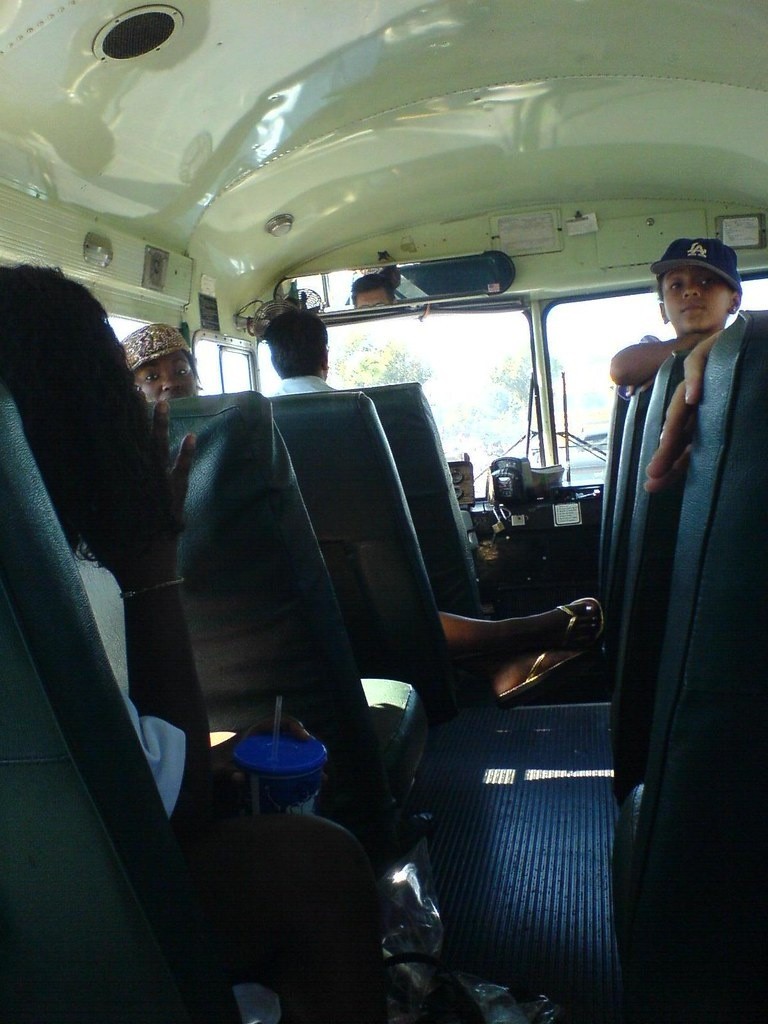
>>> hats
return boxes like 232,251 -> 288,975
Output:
650,238 -> 742,296
118,322 -> 191,373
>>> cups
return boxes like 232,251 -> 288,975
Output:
234,730 -> 327,816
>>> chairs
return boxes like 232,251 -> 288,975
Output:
0,379 -> 489,1024
594,315 -> 768,1024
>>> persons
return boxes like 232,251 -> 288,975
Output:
611,238 -> 743,493
351,274 -> 395,309
0,266 -> 389,1024
260,310 -> 335,399
119,323 -> 604,704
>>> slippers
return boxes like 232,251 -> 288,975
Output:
497,598 -> 604,703
